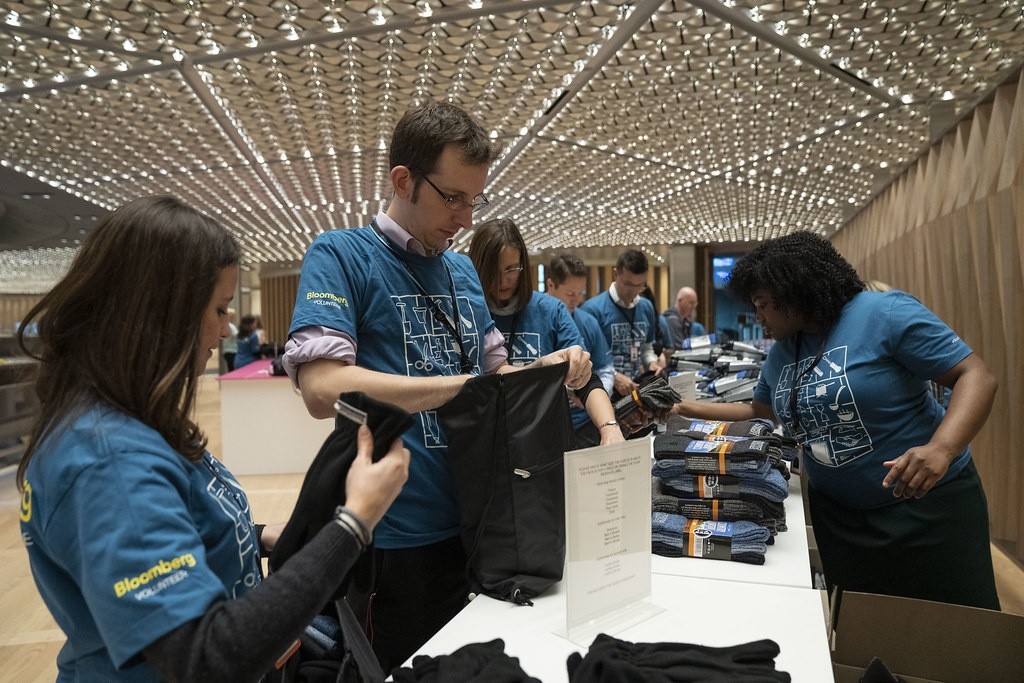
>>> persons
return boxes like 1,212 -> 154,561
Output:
221,308 -> 239,373
638,230 -> 1004,612
468,217 -> 626,446
280,98 -> 592,683
639,283 -> 673,366
663,285 -> 697,375
580,248 -> 666,396
686,311 -> 707,340
538,253 -> 617,449
10,192 -> 409,683
233,314 -> 284,370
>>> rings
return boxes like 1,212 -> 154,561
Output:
580,374 -> 585,379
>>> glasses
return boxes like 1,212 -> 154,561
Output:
415,165 -> 489,211
618,270 -> 647,291
499,262 -> 523,274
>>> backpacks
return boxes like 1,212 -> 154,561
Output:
435,359 -> 579,607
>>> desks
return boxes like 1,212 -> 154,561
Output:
387,471 -> 835,683
215,358 -> 335,475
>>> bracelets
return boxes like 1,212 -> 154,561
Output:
597,419 -> 620,428
332,505 -> 376,550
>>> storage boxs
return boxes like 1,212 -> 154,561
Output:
833,591 -> 1024,683
